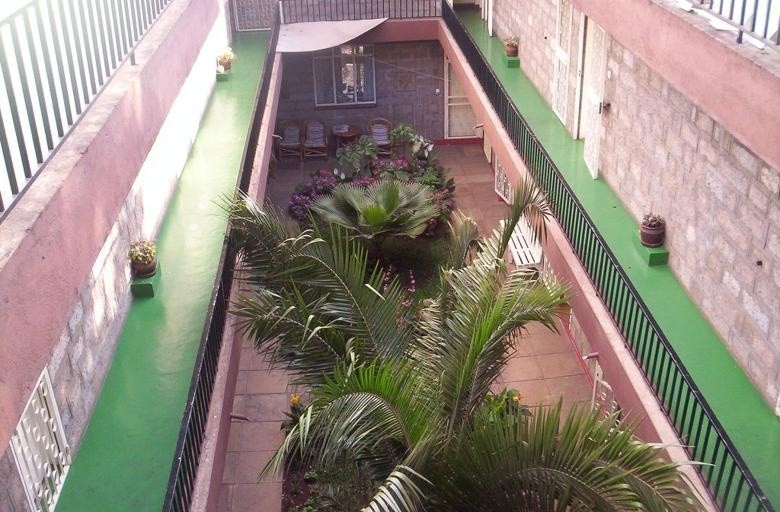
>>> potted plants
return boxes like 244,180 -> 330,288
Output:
639,212 -> 666,248
504,36 -> 519,57
128,240 -> 158,278
217,48 -> 235,70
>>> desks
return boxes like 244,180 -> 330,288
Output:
333,123 -> 362,157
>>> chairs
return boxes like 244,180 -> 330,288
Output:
302,120 -> 329,160
278,120 -> 302,160
368,117 -> 394,160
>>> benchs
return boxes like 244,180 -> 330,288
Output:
499,217 -> 543,270
